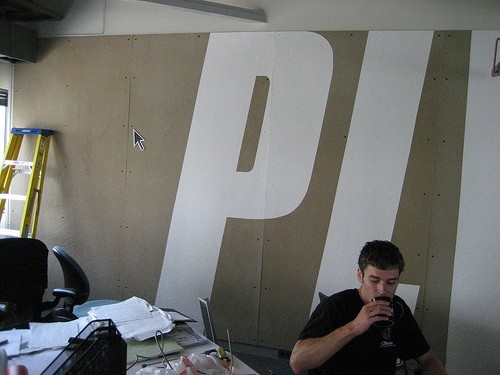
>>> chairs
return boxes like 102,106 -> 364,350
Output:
0,237 -> 77,331
199,297 -> 216,343
52,246 -> 121,318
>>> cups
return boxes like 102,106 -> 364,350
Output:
372,297 -> 394,327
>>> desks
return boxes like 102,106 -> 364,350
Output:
0,323 -> 259,375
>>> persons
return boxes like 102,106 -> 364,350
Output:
290,239 -> 448,375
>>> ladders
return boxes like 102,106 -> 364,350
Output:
0,128 -> 55,238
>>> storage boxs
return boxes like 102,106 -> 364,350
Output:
39,320 -> 128,374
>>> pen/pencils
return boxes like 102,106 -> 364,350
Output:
0,339 -> 8,345
